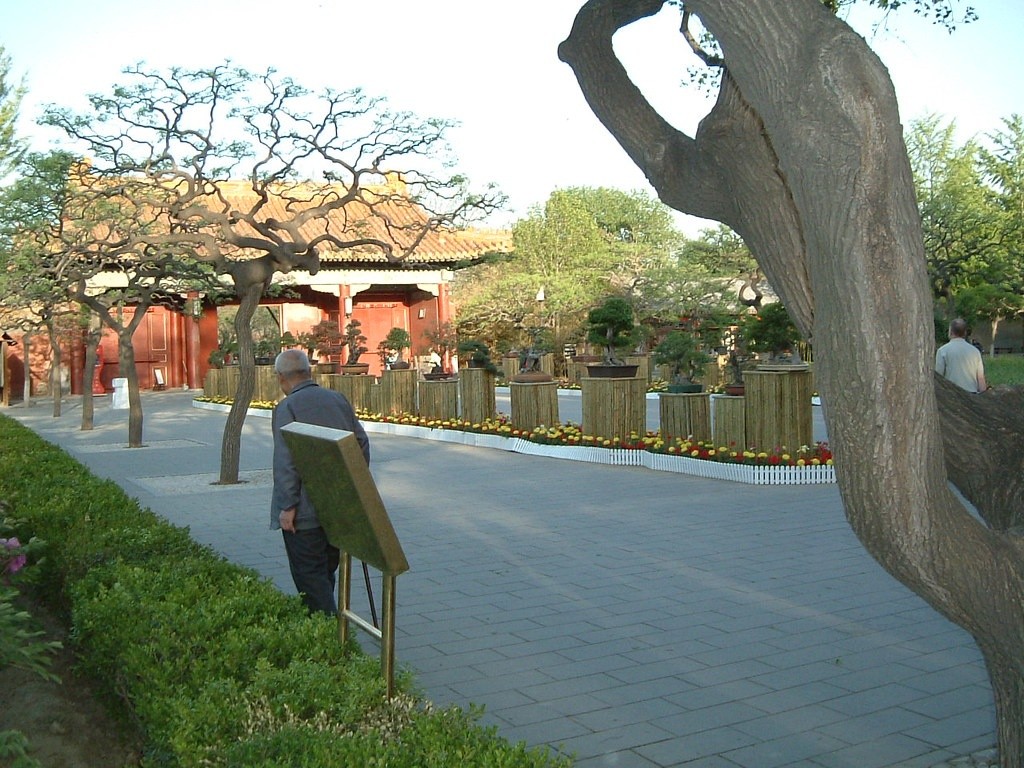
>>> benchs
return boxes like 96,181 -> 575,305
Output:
994,346 -> 1012,354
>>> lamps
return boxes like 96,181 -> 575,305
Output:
192,295 -> 201,320
344,297 -> 354,318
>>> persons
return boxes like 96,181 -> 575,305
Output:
224,349 -> 239,364
269,349 -> 370,621
935,318 -> 986,393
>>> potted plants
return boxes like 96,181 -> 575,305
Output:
388,328 -> 412,369
581,300 -> 655,377
510,325 -> 559,382
741,303 -> 810,373
463,339 -> 505,377
726,353 -> 745,395
210,322 -> 370,376
653,331 -> 709,393
420,328 -> 451,380
